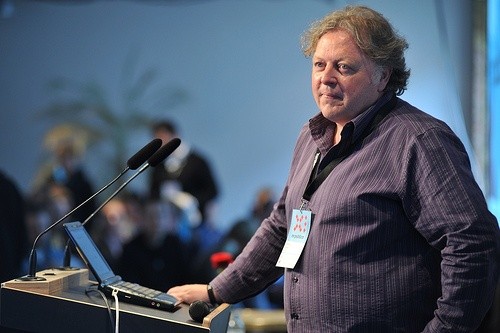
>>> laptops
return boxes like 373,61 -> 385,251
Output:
63,222 -> 184,311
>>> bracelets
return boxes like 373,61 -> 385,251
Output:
207,285 -> 218,306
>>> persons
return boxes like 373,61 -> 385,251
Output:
1,170 -> 292,310
25,124 -> 100,236
166,4 -> 499,333
148,118 -> 216,232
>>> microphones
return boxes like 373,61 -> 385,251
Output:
21,138 -> 163,281
188,300 -> 218,323
57,138 -> 182,271
